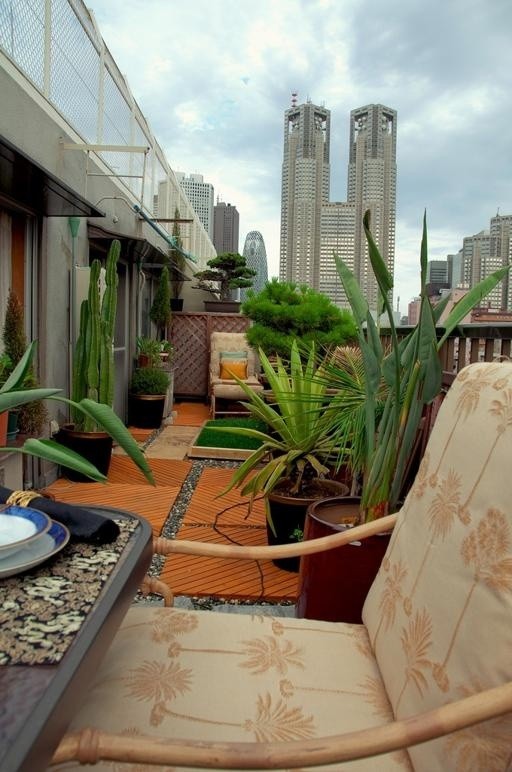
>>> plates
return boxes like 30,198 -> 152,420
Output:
0,518 -> 71,579
0,503 -> 52,561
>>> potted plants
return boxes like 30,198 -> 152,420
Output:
191,252 -> 258,312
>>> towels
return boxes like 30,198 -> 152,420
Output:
0,484 -> 121,548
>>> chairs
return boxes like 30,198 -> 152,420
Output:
38,353 -> 512,771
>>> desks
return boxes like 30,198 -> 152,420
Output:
0,489 -> 155,772
